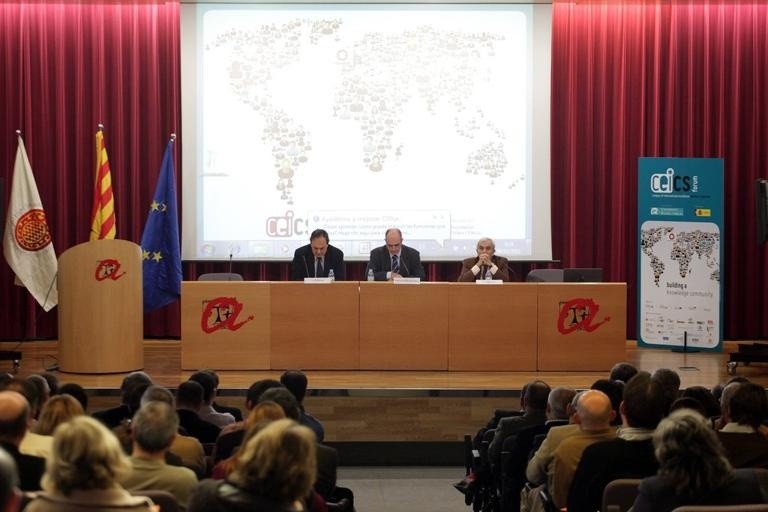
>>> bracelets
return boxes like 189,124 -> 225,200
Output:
490,263 -> 494,268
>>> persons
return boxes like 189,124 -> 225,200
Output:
565,371 -> 671,408
166,414 -> 199,477
728,376 -> 751,382
21,414 -> 161,512
122,400 -> 199,414
121,400 -> 199,512
46,390 -> 121,414
628,408 -> 768,512
92,415 -> 121,444
121,390 -> 199,400
611,363 -> 670,371
712,382 -> 751,407
319,422 -> 354,512
671,370 -> 712,408
21,414 -> 46,458
756,422 -> 768,434
565,371 -> 671,511
199,418 -> 274,480
713,408 -> 726,430
456,237 -> 511,283
290,229 -> 348,282
121,420 -> 132,456
295,418 -> 318,512
363,228 -> 426,281
0,390 -> 46,492
198,390 -> 315,418
712,382 -> 768,469
46,414 -> 83,451
187,418 -> 319,512
564,408 -> 623,510
1,369 -> 308,389
18,390 -> 46,414
454,380 -> 565,512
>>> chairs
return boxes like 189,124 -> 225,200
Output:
199,271 -> 247,283
528,267 -> 570,285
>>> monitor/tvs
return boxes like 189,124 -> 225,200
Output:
564,268 -> 604,282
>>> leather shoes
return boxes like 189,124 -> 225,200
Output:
454,474 -> 477,494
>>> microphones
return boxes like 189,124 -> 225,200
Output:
297,249 -> 311,278
90,229 -> 106,240
229,254 -> 232,281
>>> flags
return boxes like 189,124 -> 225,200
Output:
0,131 -> 61,313
137,131 -> 183,313
85,121 -> 117,242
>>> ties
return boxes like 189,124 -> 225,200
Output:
316,257 -> 323,276
391,255 -> 399,272
482,265 -> 487,279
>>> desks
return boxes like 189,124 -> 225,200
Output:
181,278 -> 626,374
